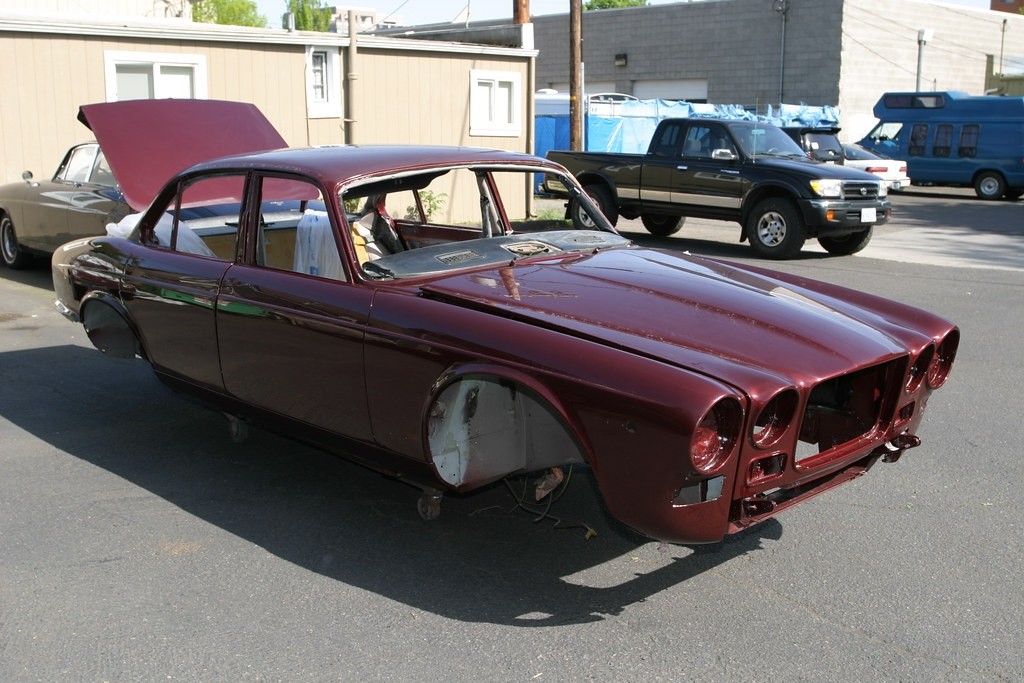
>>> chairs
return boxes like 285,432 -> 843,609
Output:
682,139 -> 703,156
717,138 -> 727,149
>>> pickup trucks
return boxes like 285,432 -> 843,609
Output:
533,113 -> 891,261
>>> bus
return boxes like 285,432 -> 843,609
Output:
855,91 -> 1024,200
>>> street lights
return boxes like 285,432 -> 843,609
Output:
916,28 -> 934,92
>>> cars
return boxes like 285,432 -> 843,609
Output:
842,142 -> 911,193
698,125 -> 845,166
0,141 -> 356,278
50,97 -> 964,558
